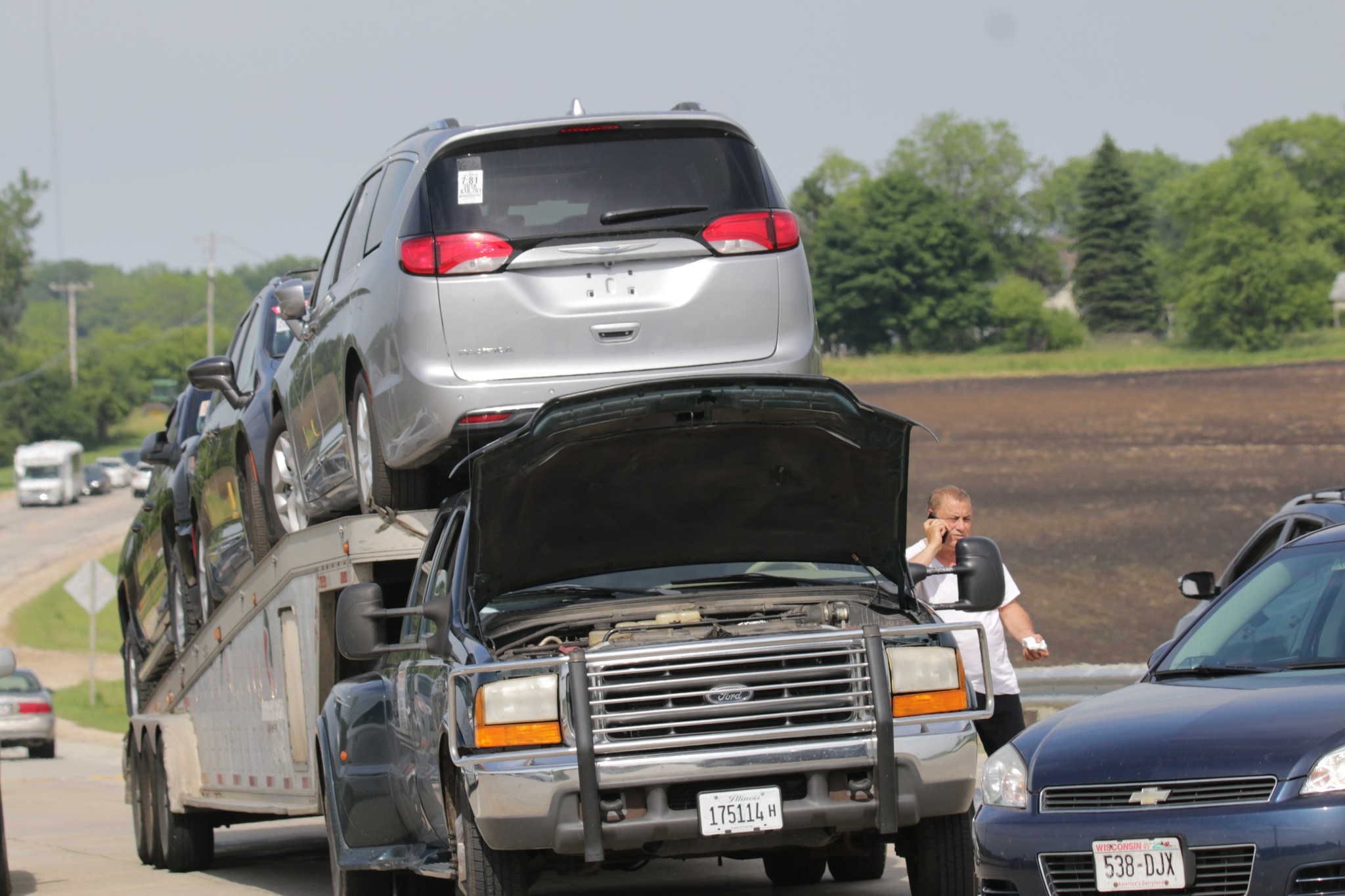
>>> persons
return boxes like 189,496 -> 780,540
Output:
905,485 -> 1049,758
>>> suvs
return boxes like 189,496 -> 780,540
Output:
312,389 -> 1009,896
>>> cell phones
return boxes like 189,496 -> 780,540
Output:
927,512 -> 949,545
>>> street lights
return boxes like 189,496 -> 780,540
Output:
48,279 -> 98,385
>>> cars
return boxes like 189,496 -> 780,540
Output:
0,669 -> 60,759
115,86 -> 825,873
80,450 -> 156,498
971,485 -> 1345,895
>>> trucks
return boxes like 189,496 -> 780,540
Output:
13,438 -> 86,506
